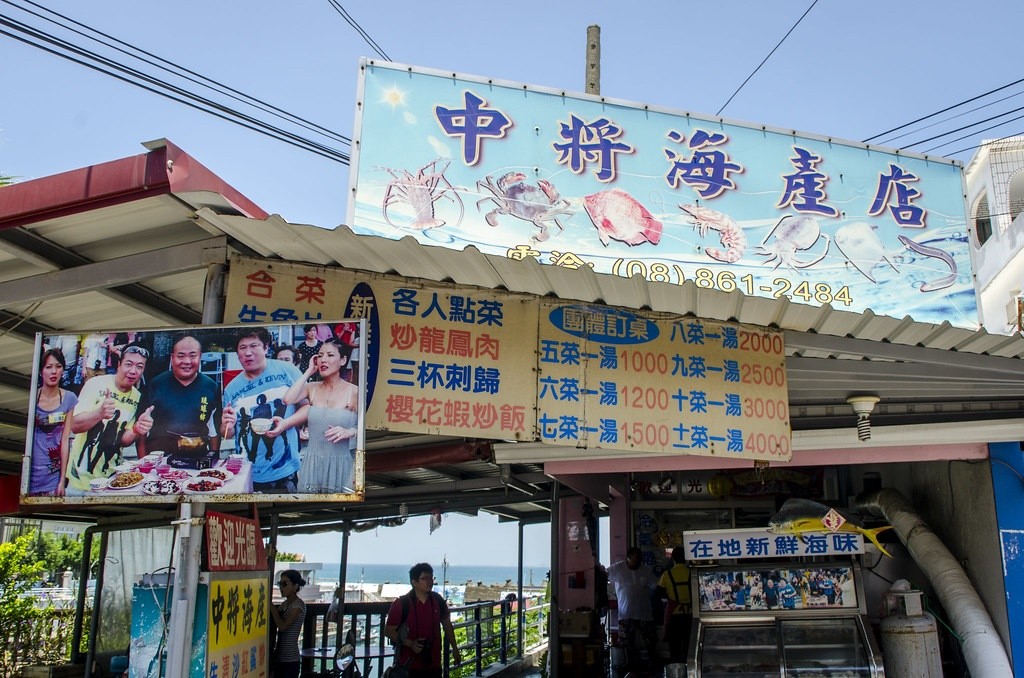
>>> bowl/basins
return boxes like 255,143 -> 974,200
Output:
90,477 -> 106,493
155,464 -> 170,474
225,453 -> 242,475
114,451 -> 164,477
250,418 -> 273,435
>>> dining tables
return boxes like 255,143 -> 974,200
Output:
86,457 -> 253,497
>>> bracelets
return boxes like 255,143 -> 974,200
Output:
350,427 -> 357,438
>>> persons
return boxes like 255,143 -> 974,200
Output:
605,547 -> 661,678
385,562 -> 461,678
64,341 -> 154,495
134,332 -> 223,471
79,331 -> 130,383
272,321 -> 357,382
26,347 -> 79,496
699,565 -> 852,610
656,545 -> 694,663
219,327 -> 312,493
270,569 -> 306,678
282,338 -> 359,494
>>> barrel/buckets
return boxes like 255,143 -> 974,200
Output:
664,664 -> 687,678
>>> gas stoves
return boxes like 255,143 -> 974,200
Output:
167,448 -> 218,470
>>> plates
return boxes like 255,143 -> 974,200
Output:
181,476 -> 225,495
158,469 -> 192,482
107,471 -> 148,489
194,467 -> 235,482
141,479 -> 182,495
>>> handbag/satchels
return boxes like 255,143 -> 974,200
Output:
383,664 -> 409,678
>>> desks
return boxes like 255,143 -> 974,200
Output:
298,646 -> 396,678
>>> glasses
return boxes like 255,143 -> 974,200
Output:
123,346 -> 149,360
418,576 -> 435,582
277,581 -> 289,587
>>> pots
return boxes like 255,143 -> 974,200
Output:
176,431 -> 211,458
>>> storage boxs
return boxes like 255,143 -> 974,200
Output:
546,612 -> 591,633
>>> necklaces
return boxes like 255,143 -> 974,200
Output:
320,378 -> 343,405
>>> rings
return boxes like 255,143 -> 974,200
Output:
337,432 -> 339,437
